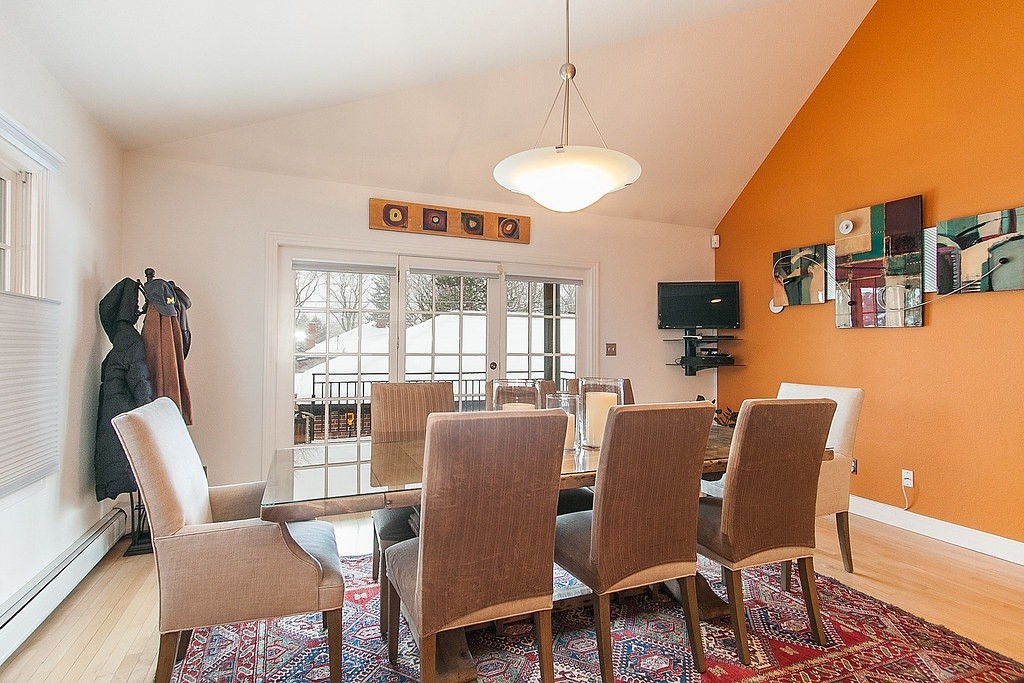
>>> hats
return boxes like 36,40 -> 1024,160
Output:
144,278 -> 177,316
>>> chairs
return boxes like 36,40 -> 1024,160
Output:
114,396 -> 347,682
485,379 -> 594,518
374,383 -> 454,581
700,382 -> 866,590
554,402 -> 716,683
388,411 -> 568,683
697,398 -> 837,665
567,378 -> 635,451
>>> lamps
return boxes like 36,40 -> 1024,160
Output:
492,1 -> 642,212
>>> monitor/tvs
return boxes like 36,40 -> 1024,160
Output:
657,281 -> 740,329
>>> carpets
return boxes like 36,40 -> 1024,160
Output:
154,552 -> 1024,683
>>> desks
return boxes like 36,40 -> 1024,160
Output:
260,409 -> 834,634
